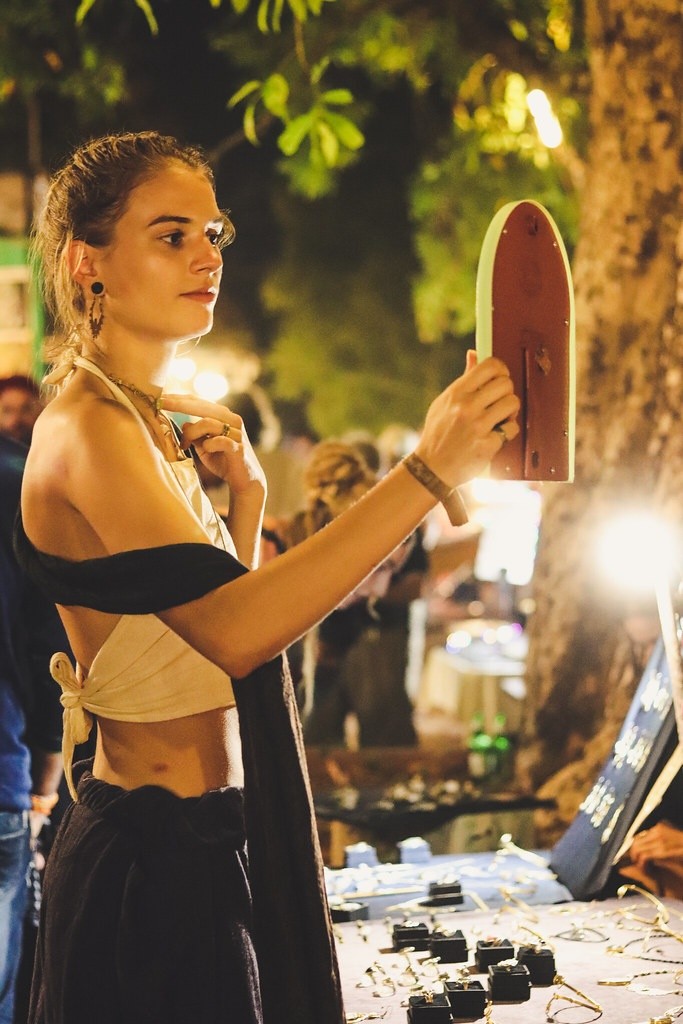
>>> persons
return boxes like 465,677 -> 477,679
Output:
547,620 -> 683,899
13,131 -> 344,1024
0,373 -> 425,1024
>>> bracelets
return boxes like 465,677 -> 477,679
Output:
403,452 -> 469,527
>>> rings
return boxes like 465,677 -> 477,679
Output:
221,423 -> 230,437
491,424 -> 507,443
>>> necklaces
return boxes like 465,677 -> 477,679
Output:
126,396 -> 226,555
108,374 -> 187,462
